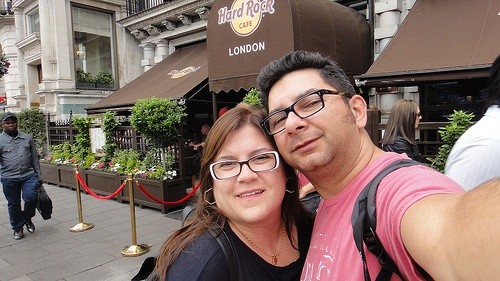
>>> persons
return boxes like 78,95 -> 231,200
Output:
382,98 -> 432,164
257,51 -> 500,281
0,112 -> 43,240
143,106 -> 316,281
444,55 -> 500,191
296,169 -> 320,216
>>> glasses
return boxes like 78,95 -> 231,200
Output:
260,89 -> 353,137
416,112 -> 420,117
210,151 -> 279,180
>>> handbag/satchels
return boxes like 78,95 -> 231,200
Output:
37,180 -> 53,220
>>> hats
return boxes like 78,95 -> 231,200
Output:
1,112 -> 17,121
219,107 -> 230,116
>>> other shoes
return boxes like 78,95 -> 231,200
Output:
11,228 -> 24,240
22,210 -> 35,233
